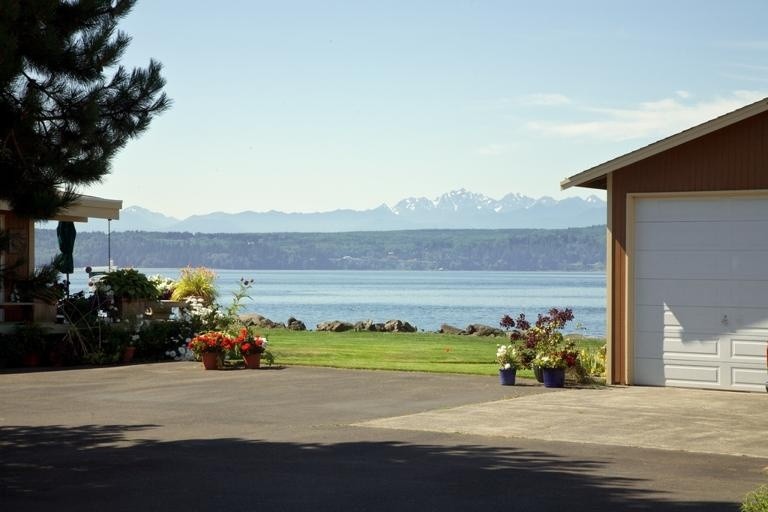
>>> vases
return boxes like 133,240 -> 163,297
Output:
242,352 -> 261,369
532,366 -> 543,384
499,367 -> 515,385
121,347 -> 136,362
201,352 -> 226,370
542,367 -> 566,387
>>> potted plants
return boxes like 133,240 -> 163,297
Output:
102,268 -> 159,316
0,252 -> 72,321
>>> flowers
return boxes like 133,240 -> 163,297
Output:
116,313 -> 150,346
496,308 -> 580,369
185,278 -> 279,367
